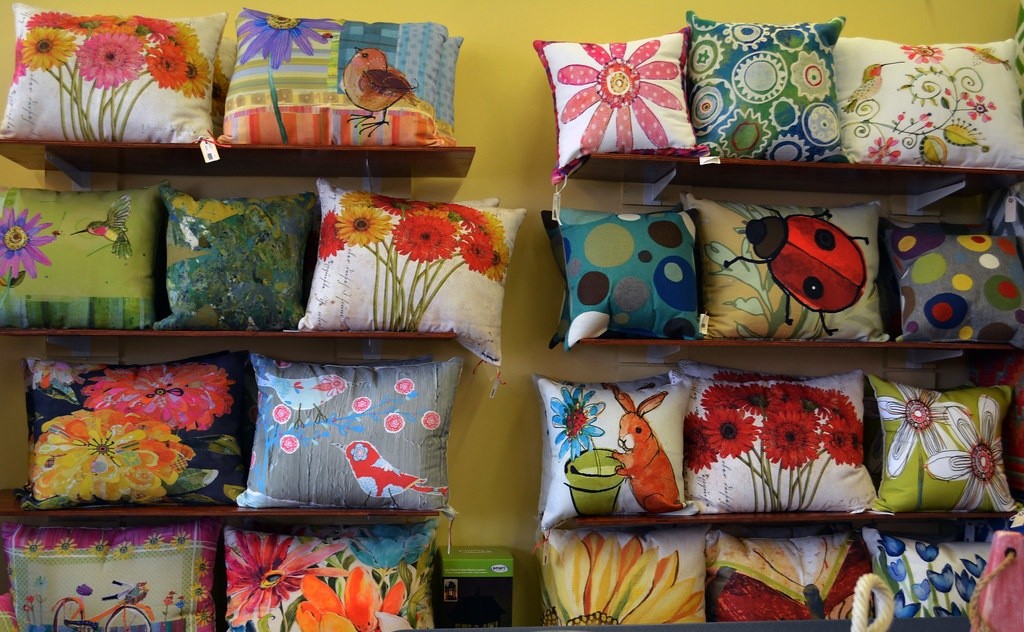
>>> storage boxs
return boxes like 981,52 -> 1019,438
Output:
436,546 -> 513,629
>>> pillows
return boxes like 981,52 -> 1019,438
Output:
152,185 -> 321,331
541,202 -> 703,351
530,373 -> 700,531
298,176 -> 528,366
532,25 -> 710,185
705,526 -> 875,621
878,217 -> 1024,349
0,177 -> 171,329
686,10 -> 849,163
12,349 -> 258,511
218,7 -> 465,147
679,190 -> 890,342
668,359 -> 878,514
0,2 -> 237,143
0,517 -> 223,632
865,372 -> 1024,514
223,518 -> 439,632
862,526 -> 992,618
235,350 -> 465,520
535,525 -> 710,626
834,37 -> 1024,171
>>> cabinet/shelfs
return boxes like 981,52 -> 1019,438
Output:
0,139 -> 475,517
568,148 -> 1024,525
